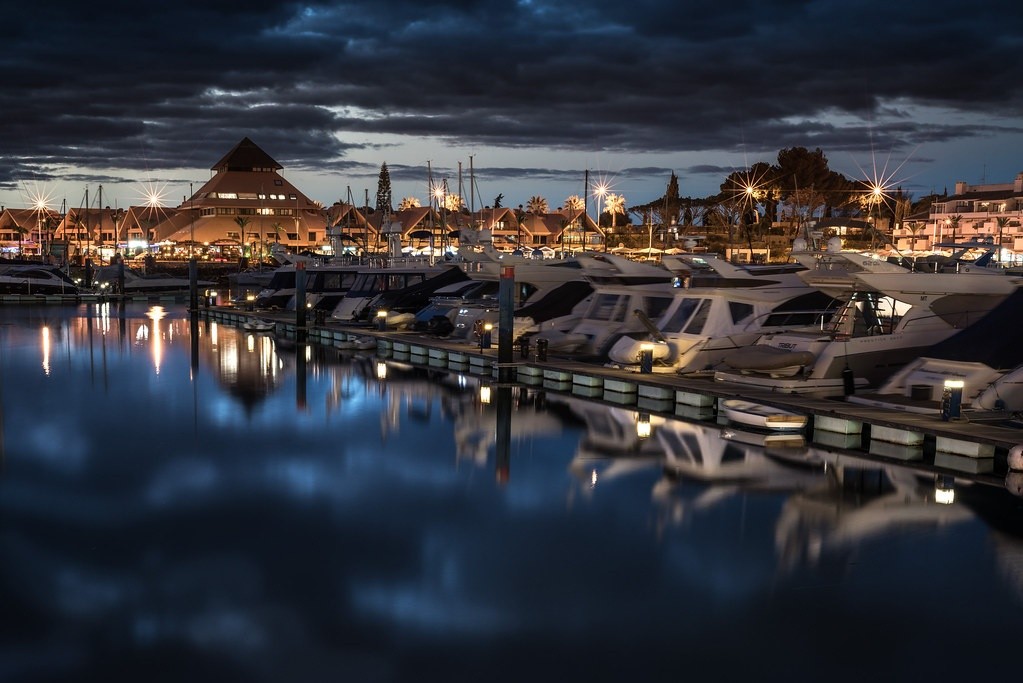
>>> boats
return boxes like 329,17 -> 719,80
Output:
0,156 -> 1021,433
564,396 -> 676,484
655,417 -> 814,498
767,462 -> 976,573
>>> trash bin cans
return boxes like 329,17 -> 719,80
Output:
313,309 -> 327,326
519,337 -> 530,358
538,338 -> 548,362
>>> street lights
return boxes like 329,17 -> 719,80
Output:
595,185 -> 606,228
870,185 -> 882,251
